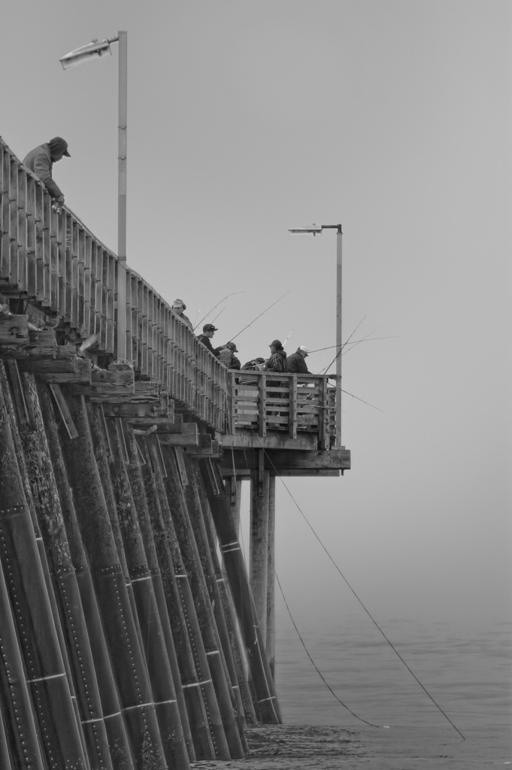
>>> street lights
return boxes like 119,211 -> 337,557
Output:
58,29 -> 134,373
290,221 -> 346,455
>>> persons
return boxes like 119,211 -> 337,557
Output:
172,299 -> 193,330
198,324 -> 225,357
223,342 -> 241,384
287,346 -> 312,374
241,358 -> 266,371
21,137 -> 71,203
252,340 -> 288,424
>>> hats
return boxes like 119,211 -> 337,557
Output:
255,358 -> 265,362
296,345 -> 307,352
172,299 -> 183,308
203,324 -> 218,332
226,342 -> 238,352
50,138 -> 70,157
270,340 -> 281,348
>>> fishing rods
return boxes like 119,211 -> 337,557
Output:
306,336 -> 394,354
328,383 -> 379,410
193,291 -> 239,332
227,300 -> 276,344
323,316 -> 367,374
